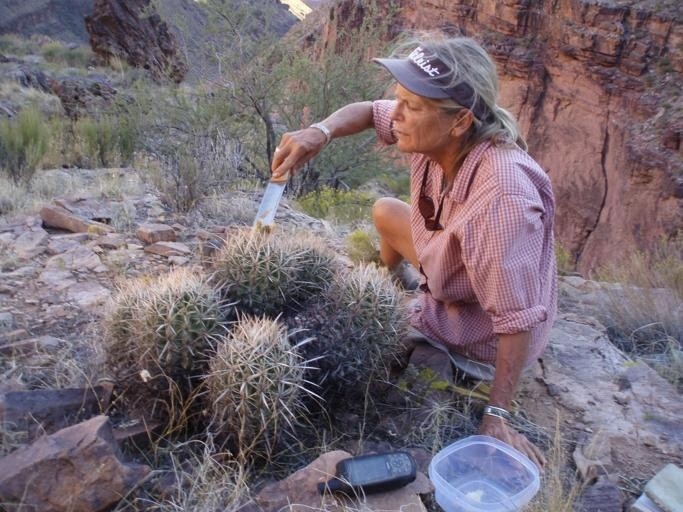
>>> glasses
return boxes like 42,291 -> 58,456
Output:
418,159 -> 447,231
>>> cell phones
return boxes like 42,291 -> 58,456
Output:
316,450 -> 416,493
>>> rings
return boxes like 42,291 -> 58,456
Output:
276,147 -> 280,152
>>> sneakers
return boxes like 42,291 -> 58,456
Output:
397,259 -> 426,291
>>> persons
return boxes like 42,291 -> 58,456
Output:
272,33 -> 560,477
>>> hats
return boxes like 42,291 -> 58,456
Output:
371,43 -> 496,125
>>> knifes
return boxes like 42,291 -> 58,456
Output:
252,166 -> 289,235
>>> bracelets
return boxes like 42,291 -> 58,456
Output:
483,405 -> 512,422
310,123 -> 331,148
